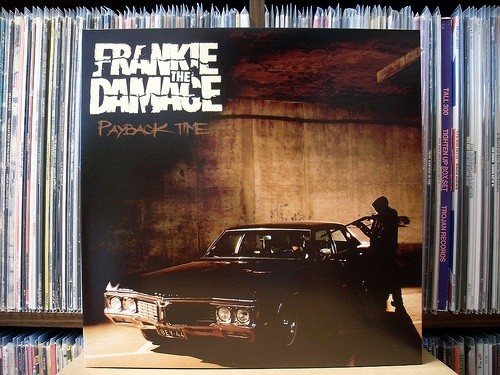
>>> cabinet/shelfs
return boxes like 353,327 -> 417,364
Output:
2,1 -> 500,375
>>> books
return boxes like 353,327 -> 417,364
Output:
1,2 -> 498,374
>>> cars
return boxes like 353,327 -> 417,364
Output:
101,221 -> 371,348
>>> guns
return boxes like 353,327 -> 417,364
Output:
321,214 -> 379,238
